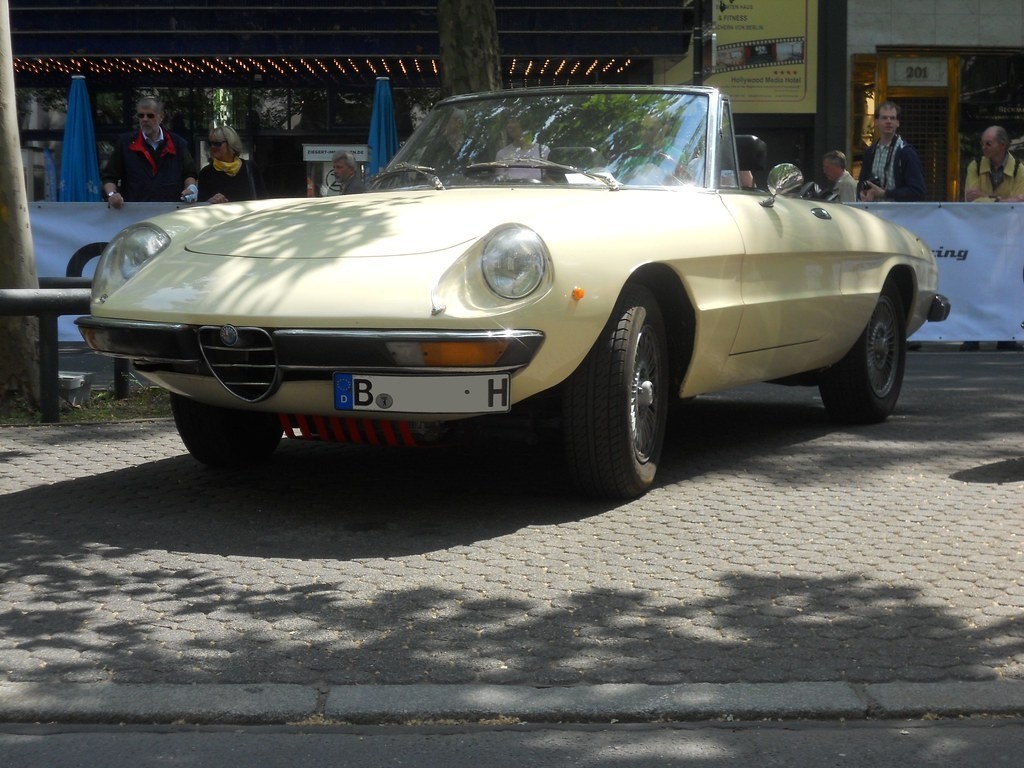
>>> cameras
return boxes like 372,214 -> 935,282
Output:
861,176 -> 880,190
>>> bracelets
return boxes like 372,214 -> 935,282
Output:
994,197 -> 1000,202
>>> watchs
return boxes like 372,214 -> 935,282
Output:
106,191 -> 118,199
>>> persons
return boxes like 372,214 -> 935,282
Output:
410,104 -> 758,192
333,152 -> 369,195
964,125 -> 1024,203
822,102 -> 929,202
101,95 -> 199,209
198,126 -> 269,204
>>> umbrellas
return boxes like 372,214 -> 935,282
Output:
367,76 -> 401,180
59,74 -> 103,202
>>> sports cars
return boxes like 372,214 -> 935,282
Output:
75,84 -> 953,502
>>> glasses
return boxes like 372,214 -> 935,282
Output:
980,140 -> 1004,147
136,111 -> 160,119
208,139 -> 227,148
